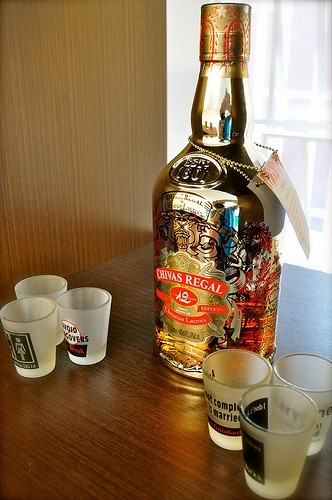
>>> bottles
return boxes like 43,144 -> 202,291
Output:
152,2 -> 282,381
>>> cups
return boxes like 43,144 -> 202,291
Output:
203,350 -> 273,452
14,275 -> 68,346
56,286 -> 112,365
1,296 -> 57,378
237,386 -> 319,500
273,353 -> 332,456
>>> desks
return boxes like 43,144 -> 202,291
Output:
0,241 -> 332,500
